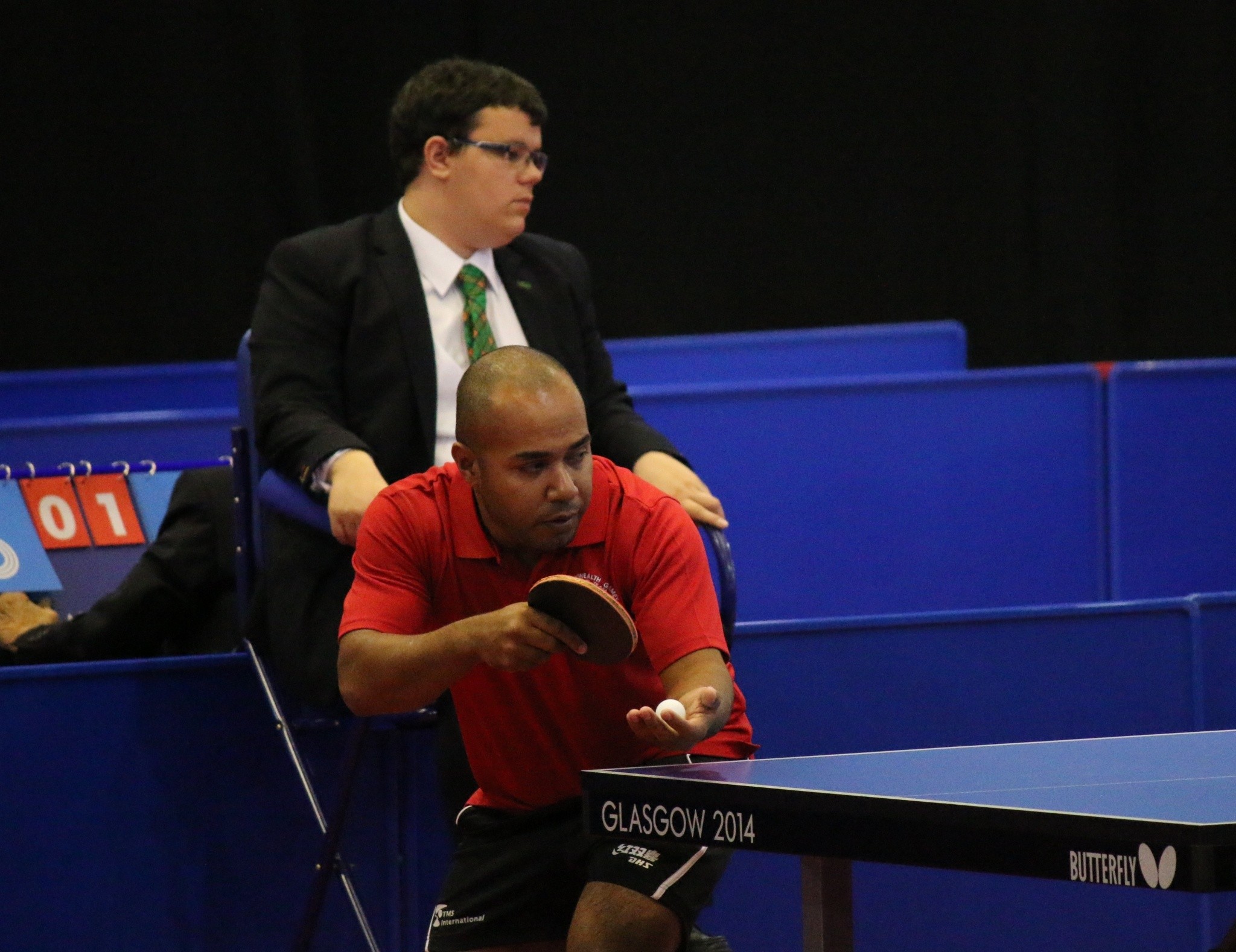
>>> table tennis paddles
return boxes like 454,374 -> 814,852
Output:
526,574 -> 638,665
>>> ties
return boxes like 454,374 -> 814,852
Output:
455,264 -> 498,364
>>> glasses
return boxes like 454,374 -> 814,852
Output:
447,133 -> 548,175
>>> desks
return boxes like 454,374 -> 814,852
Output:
583,731 -> 1236,952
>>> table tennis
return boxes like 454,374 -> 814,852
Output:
655,699 -> 686,731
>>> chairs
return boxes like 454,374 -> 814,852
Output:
228,311 -> 738,952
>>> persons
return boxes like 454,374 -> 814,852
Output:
336,347 -> 761,952
0,463 -> 233,668
248,57 -> 729,806
